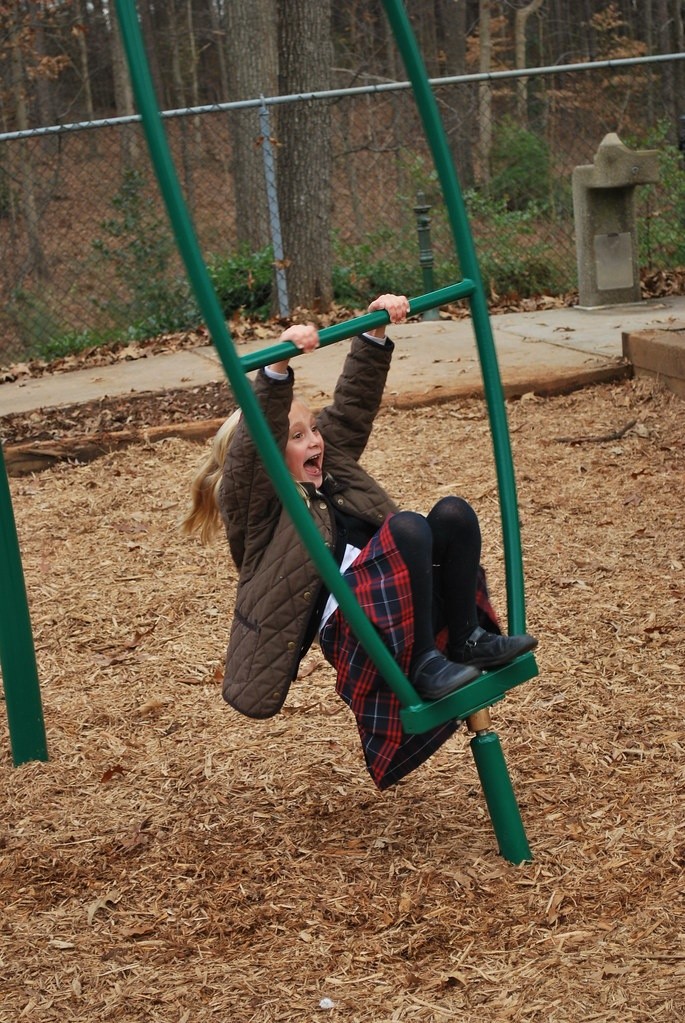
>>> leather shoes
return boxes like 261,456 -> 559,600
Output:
446,626 -> 539,668
411,649 -> 481,700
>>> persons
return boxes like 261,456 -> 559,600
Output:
174,292 -> 539,794
